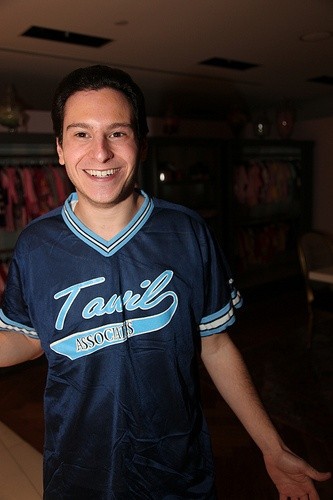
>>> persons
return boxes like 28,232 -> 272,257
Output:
1,65 -> 333,500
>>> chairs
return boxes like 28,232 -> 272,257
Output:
297,231 -> 333,348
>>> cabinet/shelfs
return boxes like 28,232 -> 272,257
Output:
144,134 -> 314,295
1,130 -> 74,263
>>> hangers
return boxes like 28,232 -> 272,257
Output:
3,161 -> 63,175
